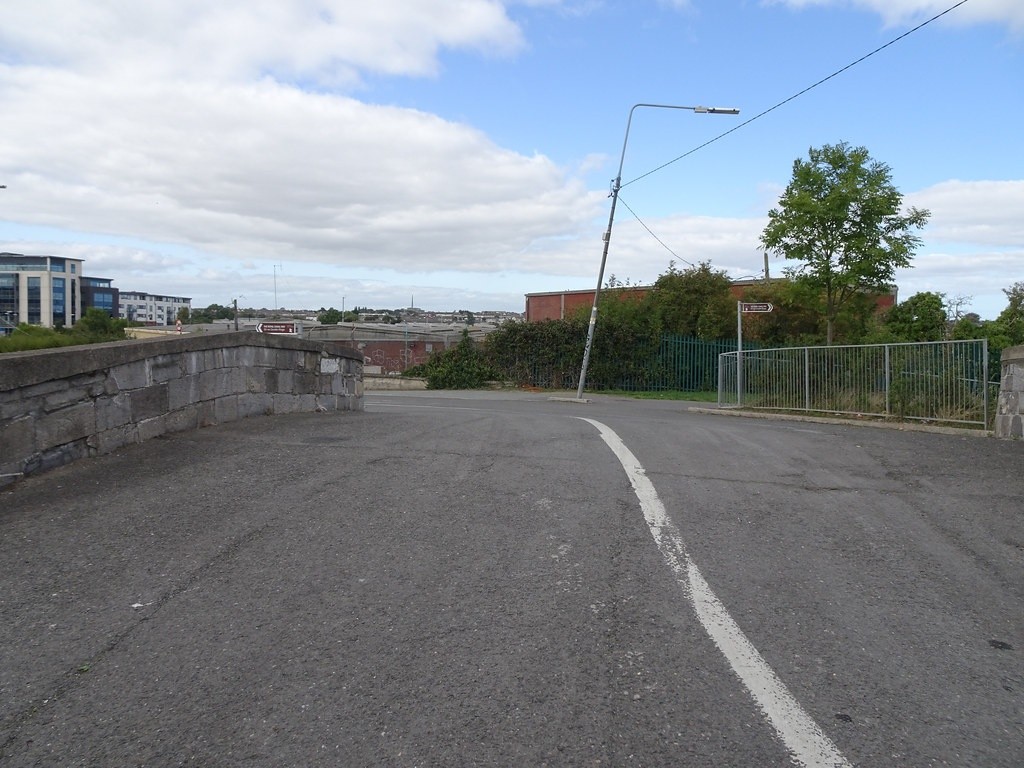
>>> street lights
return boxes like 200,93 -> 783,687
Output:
568,105 -> 741,401
